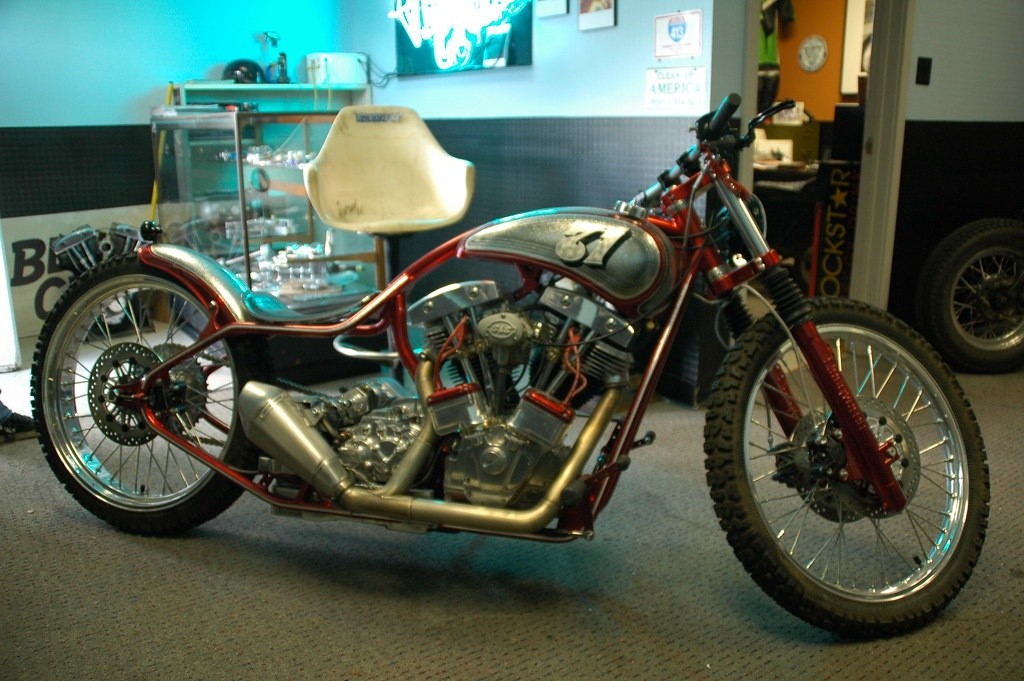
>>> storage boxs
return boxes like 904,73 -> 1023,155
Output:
756,122 -> 820,162
306,52 -> 369,83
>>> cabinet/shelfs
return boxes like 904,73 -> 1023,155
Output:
151,84 -> 389,312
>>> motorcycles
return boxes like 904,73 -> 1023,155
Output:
29,91 -> 991,642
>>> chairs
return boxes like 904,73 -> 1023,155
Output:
304,106 -> 476,385
